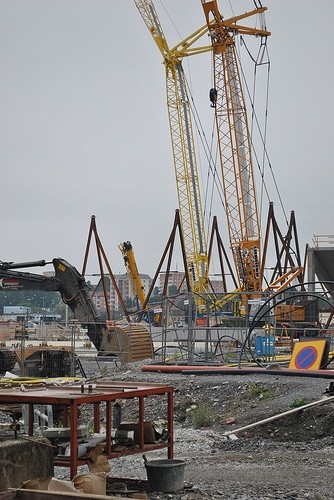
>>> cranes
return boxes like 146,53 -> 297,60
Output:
133,0 -> 213,320
120,242 -> 150,315
202,0 -> 279,321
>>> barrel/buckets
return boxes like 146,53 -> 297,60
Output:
144,458 -> 187,493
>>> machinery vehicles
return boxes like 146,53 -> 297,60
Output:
0,258 -> 158,366
